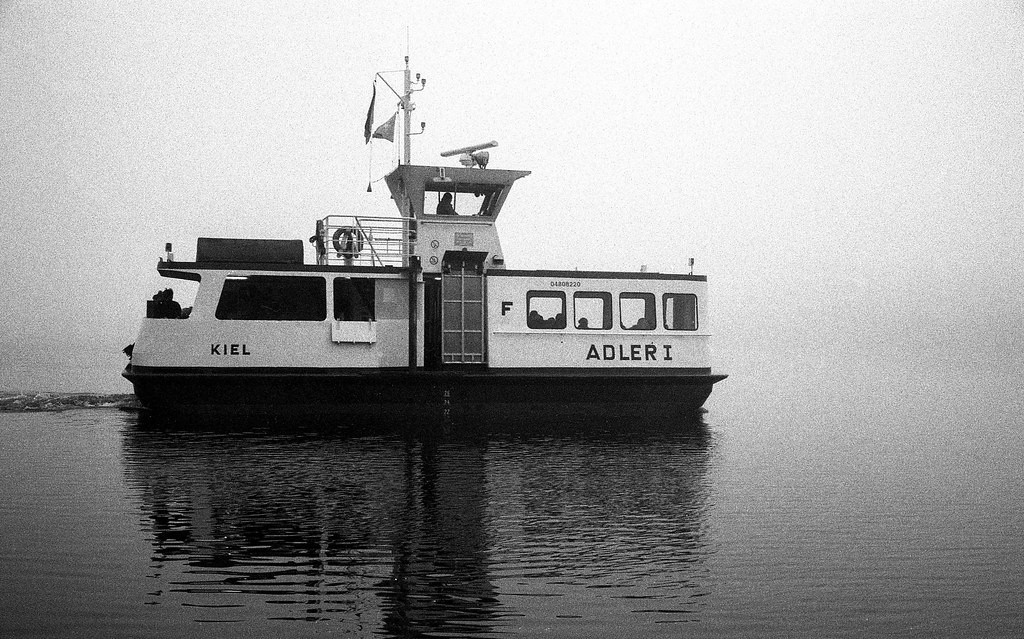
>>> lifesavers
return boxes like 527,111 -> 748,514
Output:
332,226 -> 364,258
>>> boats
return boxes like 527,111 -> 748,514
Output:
121,24 -> 731,413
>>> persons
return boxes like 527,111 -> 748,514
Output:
528,309 -> 564,328
628,317 -> 651,330
578,318 -> 589,329
436,192 -> 459,214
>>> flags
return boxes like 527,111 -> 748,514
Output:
372,112 -> 396,142
364,84 -> 376,144
